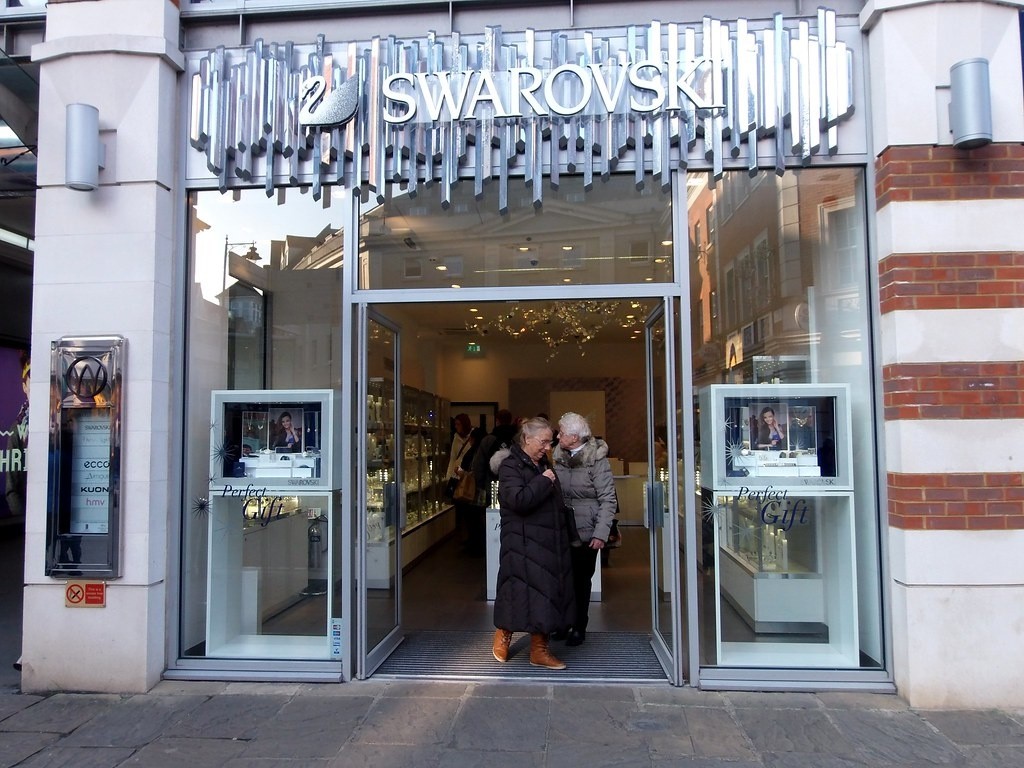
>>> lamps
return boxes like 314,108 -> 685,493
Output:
66,103 -> 105,190
948,57 -> 992,150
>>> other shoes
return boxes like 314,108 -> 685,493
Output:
465,550 -> 477,557
448,531 -> 464,548
462,539 -> 471,546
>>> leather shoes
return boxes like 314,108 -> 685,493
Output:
550,629 -> 586,646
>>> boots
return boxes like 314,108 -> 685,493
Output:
492,629 -> 513,662
529,633 -> 566,669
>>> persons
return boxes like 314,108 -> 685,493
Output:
272,411 -> 301,450
755,407 -> 784,450
6,351 -> 31,514
243,444 -> 252,457
445,409 -> 518,559
490,412 -> 617,669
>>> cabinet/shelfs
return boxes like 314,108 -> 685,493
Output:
367,378 -> 458,589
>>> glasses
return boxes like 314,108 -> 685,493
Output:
535,438 -> 553,445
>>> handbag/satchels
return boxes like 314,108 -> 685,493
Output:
446,477 -> 458,495
605,518 -> 623,548
453,470 -> 486,507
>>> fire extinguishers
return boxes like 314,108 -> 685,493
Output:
308,514 -> 328,568
